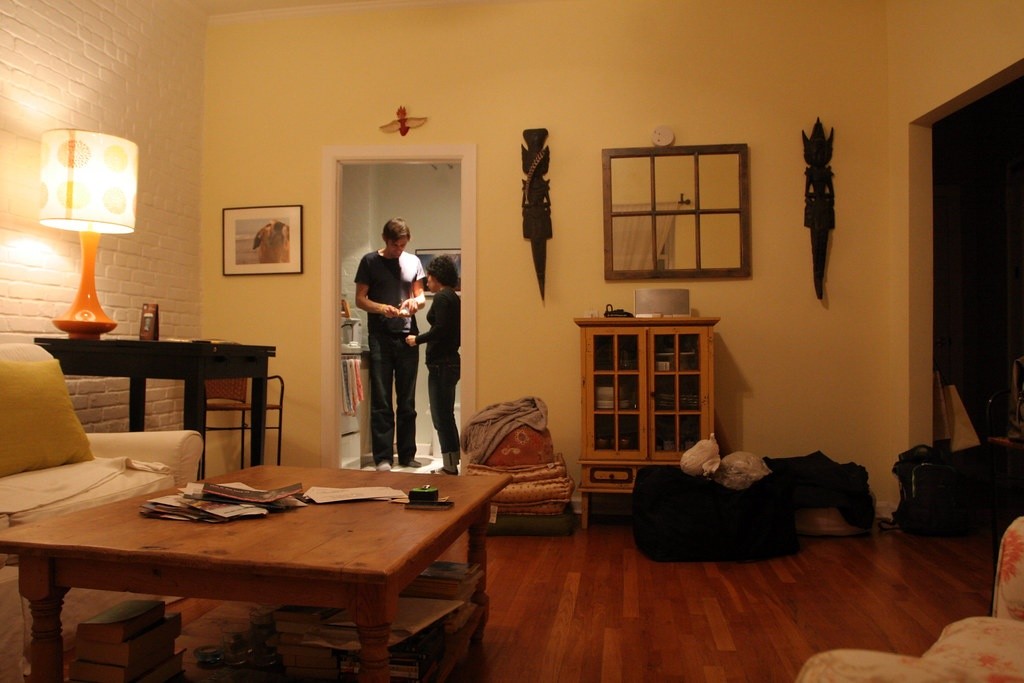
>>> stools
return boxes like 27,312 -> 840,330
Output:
207,339 -> 286,479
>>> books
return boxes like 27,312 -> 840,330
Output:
272,557 -> 484,683
68,598 -> 188,683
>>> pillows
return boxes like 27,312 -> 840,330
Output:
0,359 -> 93,478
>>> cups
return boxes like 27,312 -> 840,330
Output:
222,632 -> 248,665
249,608 -> 278,665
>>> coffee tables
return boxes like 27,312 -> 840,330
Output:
1,462 -> 512,682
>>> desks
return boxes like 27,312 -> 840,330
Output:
33,336 -> 279,480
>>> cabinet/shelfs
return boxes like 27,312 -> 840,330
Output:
575,314 -> 722,529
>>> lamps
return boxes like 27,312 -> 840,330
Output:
38,130 -> 140,337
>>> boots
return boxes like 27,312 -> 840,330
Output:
430,451 -> 459,475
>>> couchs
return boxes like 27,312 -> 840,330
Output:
0,342 -> 203,533
792,522 -> 1024,682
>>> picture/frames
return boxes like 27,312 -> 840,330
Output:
413,248 -> 463,296
223,205 -> 304,276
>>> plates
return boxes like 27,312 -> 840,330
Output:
597,388 -> 632,408
656,393 -> 699,410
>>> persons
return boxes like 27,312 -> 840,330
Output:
405,255 -> 461,477
354,217 -> 426,471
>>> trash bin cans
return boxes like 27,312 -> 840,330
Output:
427,404 -> 460,458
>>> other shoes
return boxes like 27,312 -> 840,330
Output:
409,460 -> 422,467
376,462 -> 391,471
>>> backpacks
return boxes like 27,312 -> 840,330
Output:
878,444 -> 975,537
